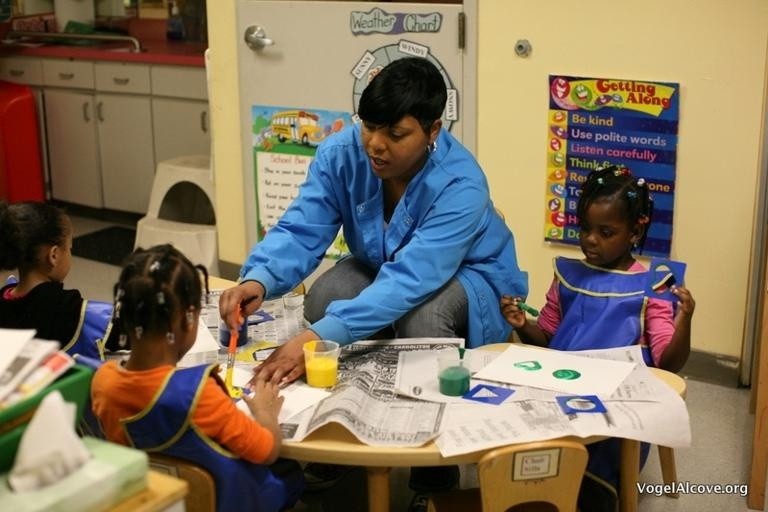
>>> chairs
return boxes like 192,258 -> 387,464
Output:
478,440 -> 589,511
143,451 -> 215,511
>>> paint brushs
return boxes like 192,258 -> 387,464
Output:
503,295 -> 539,317
225,304 -> 243,399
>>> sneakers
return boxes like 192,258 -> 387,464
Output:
406,493 -> 433,511
301,463 -> 355,496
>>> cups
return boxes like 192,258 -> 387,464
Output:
302,339 -> 340,389
219,322 -> 247,347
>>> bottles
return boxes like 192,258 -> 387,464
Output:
168,2 -> 186,43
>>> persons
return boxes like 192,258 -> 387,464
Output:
91,241 -> 306,512
218,57 -> 528,512
497,164 -> 695,512
0,200 -> 113,355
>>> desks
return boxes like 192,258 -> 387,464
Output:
116,288 -> 687,511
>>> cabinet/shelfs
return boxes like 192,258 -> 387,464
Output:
0,58 -> 51,203
43,61 -> 158,215
149,64 -> 214,171
